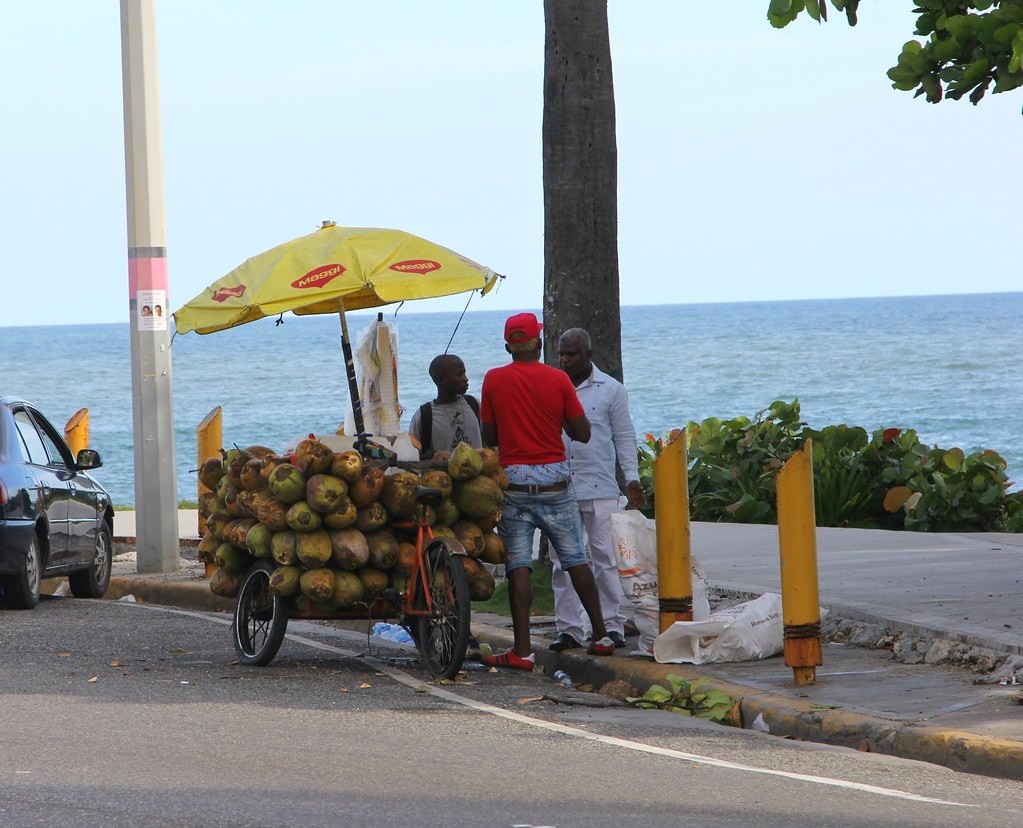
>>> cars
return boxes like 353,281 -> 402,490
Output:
0,394 -> 115,613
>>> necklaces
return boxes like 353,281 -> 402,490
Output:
440,396 -> 466,436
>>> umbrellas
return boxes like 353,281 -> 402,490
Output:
172,220 -> 505,457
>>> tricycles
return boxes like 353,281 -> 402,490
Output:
232,484 -> 471,682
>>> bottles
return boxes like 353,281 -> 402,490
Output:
554,670 -> 572,686
392,430 -> 420,463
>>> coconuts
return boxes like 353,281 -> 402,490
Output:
197,438 -> 506,616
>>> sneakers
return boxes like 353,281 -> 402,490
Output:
481,647 -> 535,673
585,637 -> 614,656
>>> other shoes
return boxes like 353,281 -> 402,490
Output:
548,633 -> 582,650
608,631 -> 625,649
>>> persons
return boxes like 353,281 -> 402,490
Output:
410,353 -> 499,660
478,313 -> 615,670
547,328 -> 648,651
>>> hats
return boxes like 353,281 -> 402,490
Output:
504,313 -> 544,344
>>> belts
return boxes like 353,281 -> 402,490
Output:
504,474 -> 571,492
